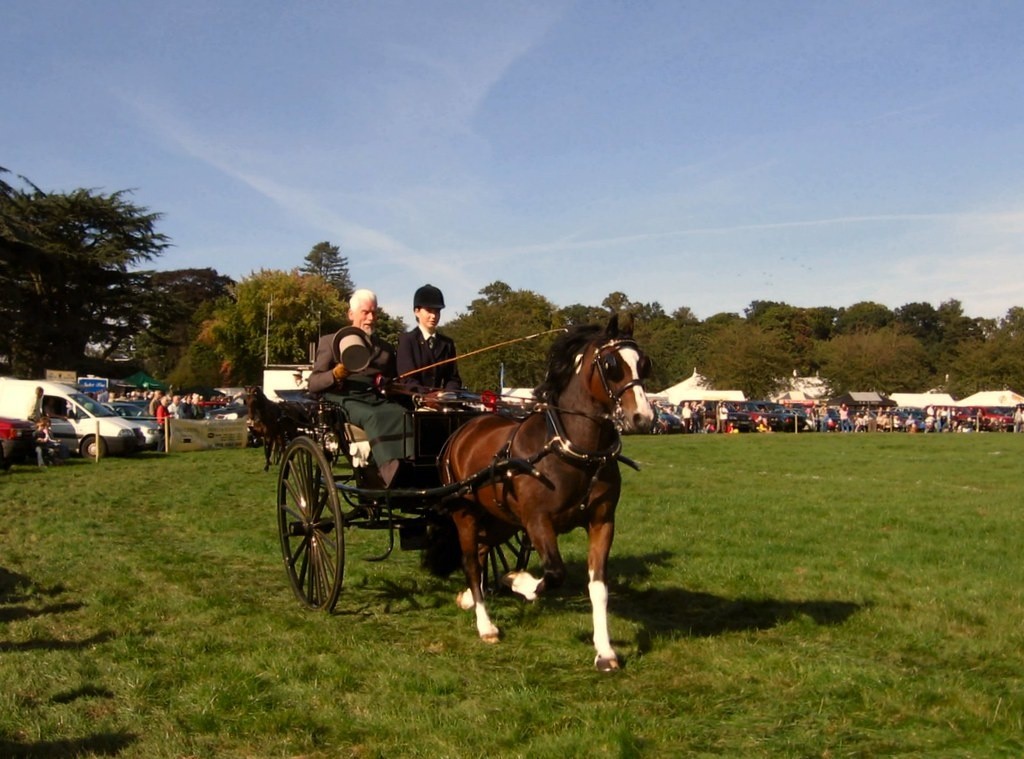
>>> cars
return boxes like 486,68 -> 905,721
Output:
657,399 -> 927,433
81,402 -> 159,449
0,417 -> 35,471
205,395 -> 250,421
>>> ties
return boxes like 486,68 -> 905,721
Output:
428,336 -> 436,352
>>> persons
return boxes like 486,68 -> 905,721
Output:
31,417 -> 67,468
646,400 -> 1024,435
44,397 -> 67,421
126,391 -> 227,452
396,284 -> 463,397
293,371 -> 306,390
307,288 -> 417,488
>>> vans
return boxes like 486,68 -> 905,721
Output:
0,376 -> 145,460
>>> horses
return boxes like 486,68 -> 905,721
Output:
419,312 -> 658,672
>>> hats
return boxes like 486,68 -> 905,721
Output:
331,326 -> 373,372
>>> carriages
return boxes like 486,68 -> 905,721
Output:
254,312 -> 656,674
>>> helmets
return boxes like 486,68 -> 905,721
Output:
413,284 -> 444,309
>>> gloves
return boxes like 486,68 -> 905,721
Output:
333,363 -> 349,379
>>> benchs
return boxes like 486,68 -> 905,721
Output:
262,369 -> 313,404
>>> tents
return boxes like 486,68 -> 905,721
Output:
123,371 -> 163,391
